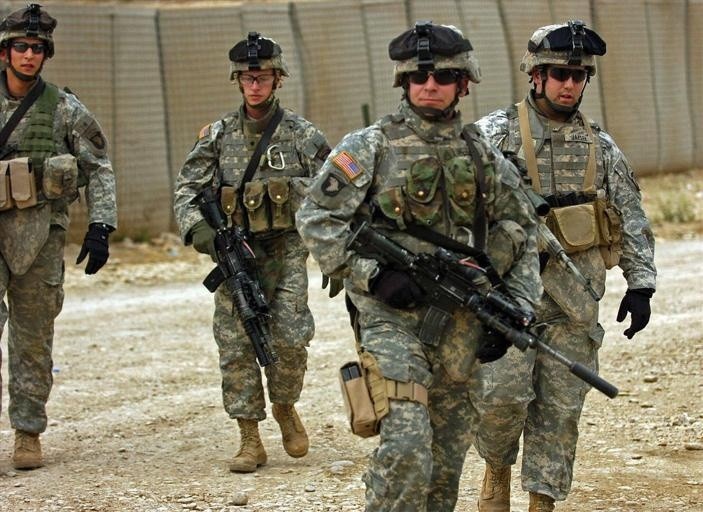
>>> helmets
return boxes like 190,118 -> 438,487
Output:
388,19 -> 483,88
227,30 -> 289,82
518,19 -> 606,76
1,3 -> 58,57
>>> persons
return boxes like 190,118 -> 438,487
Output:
292,20 -> 543,510
0,2 -> 119,470
171,32 -> 348,474
469,21 -> 657,509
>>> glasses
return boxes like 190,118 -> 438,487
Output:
11,41 -> 48,53
406,69 -> 466,86
238,73 -> 275,86
547,66 -> 588,82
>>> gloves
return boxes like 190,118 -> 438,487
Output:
616,287 -> 654,340
75,223 -> 110,275
370,263 -> 426,310
475,326 -> 509,364
189,219 -> 220,264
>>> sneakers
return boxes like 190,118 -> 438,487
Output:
228,419 -> 267,474
477,462 -> 511,512
12,431 -> 42,471
271,402 -> 309,459
527,491 -> 555,512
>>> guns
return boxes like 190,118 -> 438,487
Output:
188,188 -> 277,365
502,149 -> 601,302
346,222 -> 617,399
